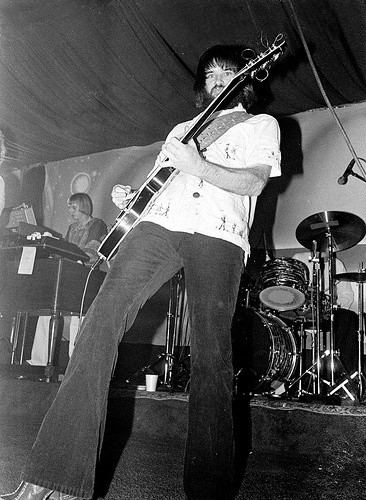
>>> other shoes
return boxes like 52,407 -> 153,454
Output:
0,479 -> 84,500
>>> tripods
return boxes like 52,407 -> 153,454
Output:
121,275 -> 192,391
288,228 -> 361,403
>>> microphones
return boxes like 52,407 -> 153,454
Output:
338,159 -> 356,185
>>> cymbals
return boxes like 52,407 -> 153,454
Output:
295,210 -> 366,252
332,272 -> 366,283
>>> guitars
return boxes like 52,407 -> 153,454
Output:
97,32 -> 289,260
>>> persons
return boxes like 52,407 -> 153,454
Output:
292,250 -> 359,372
24,193 -> 108,369
0,45 -> 282,500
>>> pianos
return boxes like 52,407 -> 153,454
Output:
0,224 -> 106,384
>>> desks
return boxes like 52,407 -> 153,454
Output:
0,257 -> 107,382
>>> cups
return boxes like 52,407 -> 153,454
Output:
145,374 -> 159,392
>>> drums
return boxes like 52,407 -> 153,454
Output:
236,257 -> 309,311
276,291 -> 329,322
231,306 -> 296,396
